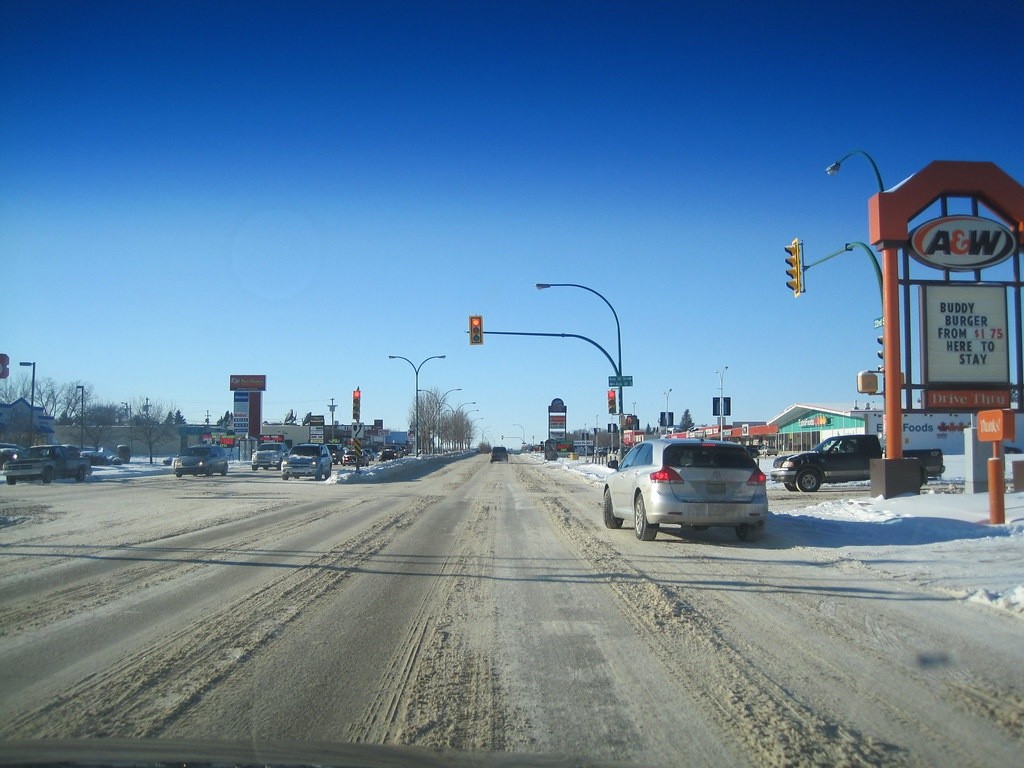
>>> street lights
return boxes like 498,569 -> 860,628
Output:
20,362 -> 36,445
535,284 -> 623,463
716,366 -> 728,441
663,389 -> 672,436
418,388 -> 483,455
389,356 -> 446,458
77,386 -> 84,449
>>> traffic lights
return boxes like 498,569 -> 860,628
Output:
352,391 -> 362,419
608,391 -> 616,413
877,336 -> 886,372
784,238 -> 801,297
469,315 -> 483,345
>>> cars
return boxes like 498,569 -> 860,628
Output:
743,445 -> 760,458
251,441 -> 408,482
489,447 -> 508,463
758,446 -> 779,456
603,439 -> 768,542
0,441 -> 103,486
172,445 -> 228,476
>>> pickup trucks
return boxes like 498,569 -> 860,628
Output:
770,435 -> 945,492
